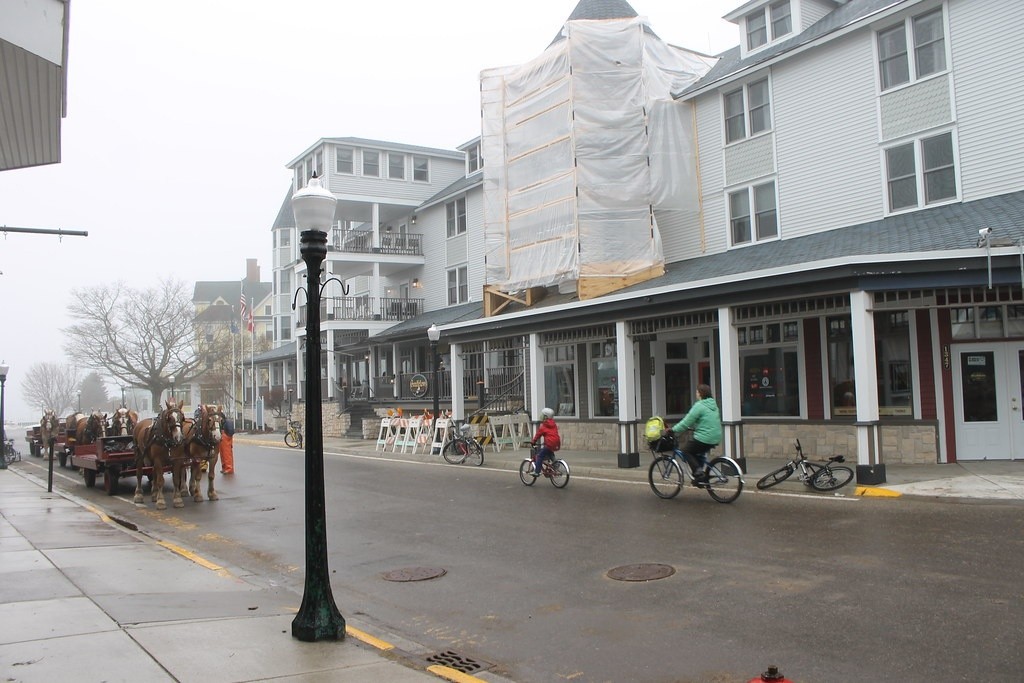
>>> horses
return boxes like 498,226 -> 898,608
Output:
177,401 -> 223,502
40,409 -> 59,461
66,406 -> 137,476
132,400 -> 185,510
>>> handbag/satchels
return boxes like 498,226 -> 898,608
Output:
649,432 -> 678,453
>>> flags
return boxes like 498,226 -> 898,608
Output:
231,305 -> 240,334
248,303 -> 256,333
240,284 -> 247,321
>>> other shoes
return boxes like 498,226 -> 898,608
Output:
690,470 -> 710,484
529,471 -> 540,476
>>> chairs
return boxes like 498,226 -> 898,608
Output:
386,302 -> 418,319
380,238 -> 420,254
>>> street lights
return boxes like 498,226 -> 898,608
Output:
77,390 -> 81,411
169,374 -> 175,397
0,360 -> 9,469
291,170 -> 348,642
121,382 -> 125,407
41,400 -> 45,417
426,323 -> 444,455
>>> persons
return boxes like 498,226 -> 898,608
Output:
528,408 -> 560,476
664,384 -> 723,488
214,410 -> 235,473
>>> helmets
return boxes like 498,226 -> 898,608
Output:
541,407 -> 555,420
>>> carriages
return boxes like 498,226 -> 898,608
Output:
54,407 -> 138,477
71,400 -> 222,510
25,409 -> 85,461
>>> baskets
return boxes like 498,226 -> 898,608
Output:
642,427 -> 679,451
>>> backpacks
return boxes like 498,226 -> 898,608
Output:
644,416 -> 665,442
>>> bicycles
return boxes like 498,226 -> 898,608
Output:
443,419 -> 484,466
646,430 -> 746,503
519,441 -> 570,488
755,438 -> 854,492
4,439 -> 16,464
284,418 -> 303,448
